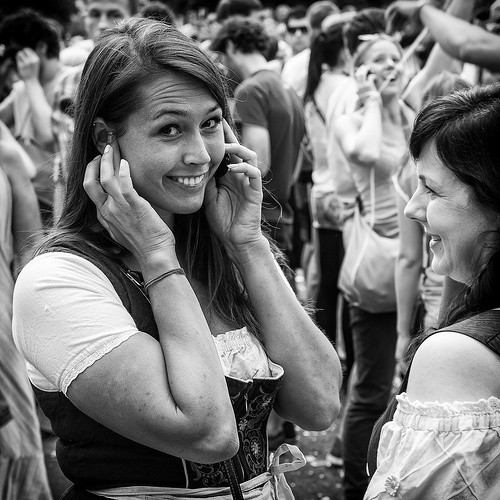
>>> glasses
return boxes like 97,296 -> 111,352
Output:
287,26 -> 308,33
2,47 -> 21,60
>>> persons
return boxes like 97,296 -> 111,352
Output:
0,0 -> 500,467
334,2 -> 474,500
11,17 -> 344,500
0,122 -> 55,500
362,81 -> 500,500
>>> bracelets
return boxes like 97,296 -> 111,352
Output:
412,0 -> 437,29
145,268 -> 186,290
